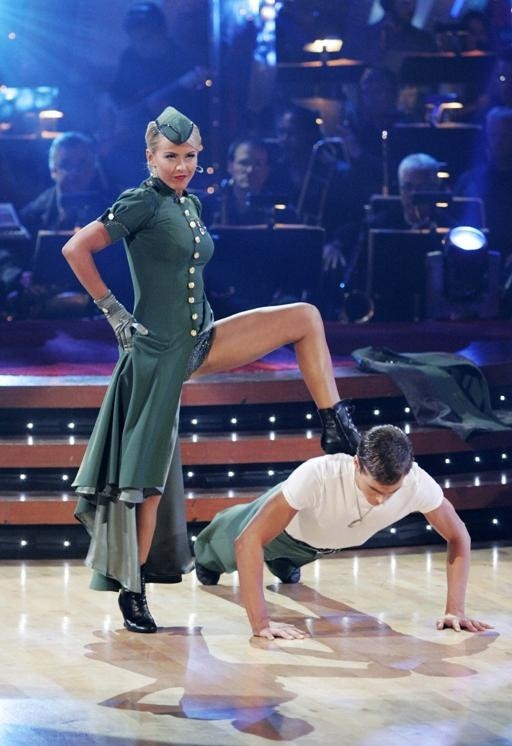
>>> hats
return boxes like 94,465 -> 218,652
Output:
126,3 -> 164,34
156,106 -> 194,144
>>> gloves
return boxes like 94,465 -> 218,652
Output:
93,288 -> 149,352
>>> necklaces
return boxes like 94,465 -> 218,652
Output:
346,491 -> 376,529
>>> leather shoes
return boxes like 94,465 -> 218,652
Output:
265,559 -> 300,584
195,561 -> 220,585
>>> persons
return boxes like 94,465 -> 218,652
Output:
61,104 -> 368,636
191,423 -> 495,643
2,0 -> 512,339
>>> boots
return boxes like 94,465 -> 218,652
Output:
118,576 -> 158,633
318,399 -> 362,457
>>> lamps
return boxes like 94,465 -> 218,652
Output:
425,225 -> 501,320
